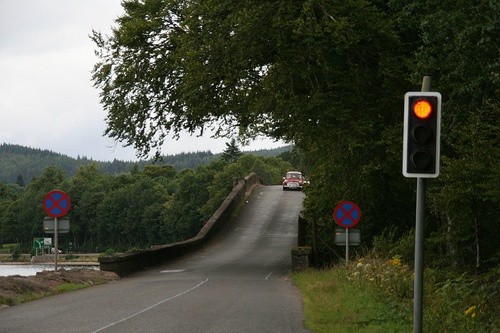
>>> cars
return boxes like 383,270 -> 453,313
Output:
282,169 -> 306,191
50,247 -> 65,255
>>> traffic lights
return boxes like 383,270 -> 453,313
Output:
402,91 -> 441,177
